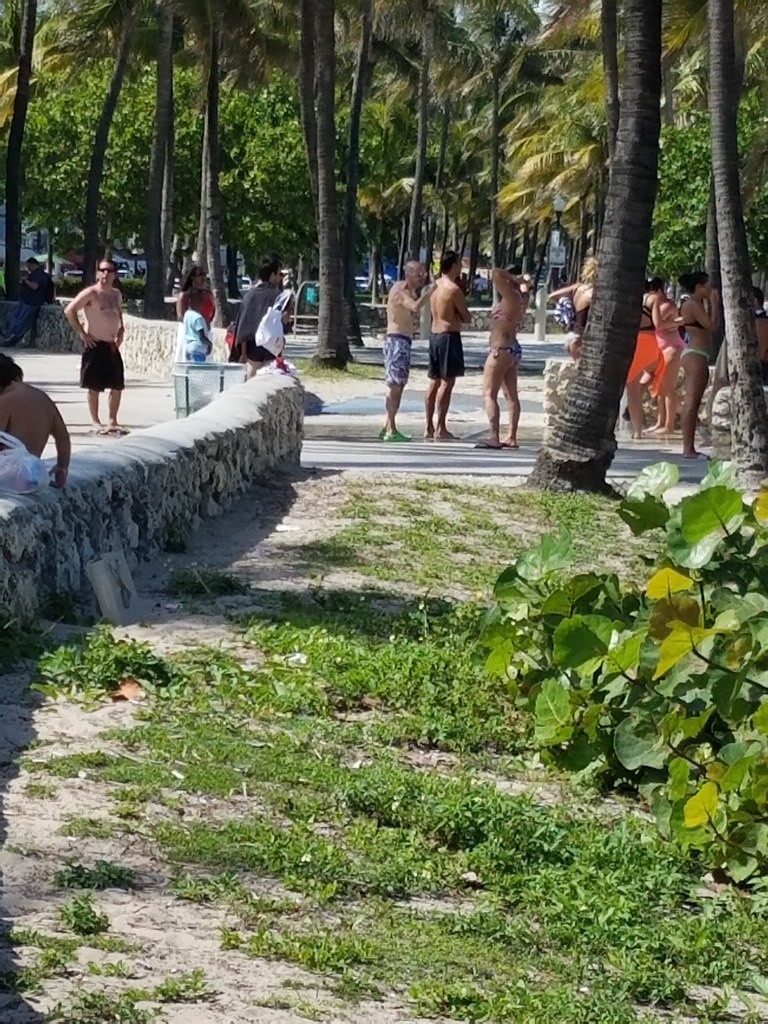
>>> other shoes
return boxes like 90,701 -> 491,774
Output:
383,430 -> 411,441
379,427 -> 386,439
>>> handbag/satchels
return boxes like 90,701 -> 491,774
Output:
255,292 -> 295,358
0,431 -> 49,494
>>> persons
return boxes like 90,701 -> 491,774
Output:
545,257 -> 597,364
0,257 -> 56,347
625,277 -> 686,441
378,260 -> 437,441
227,253 -> 296,384
181,286 -> 213,362
679,271 -> 720,462
751,286 -> 768,387
174,265 -> 216,365
474,267 -> 534,449
0,353 -> 72,489
64,259 -> 131,434
423,250 -> 471,441
642,277 -> 685,436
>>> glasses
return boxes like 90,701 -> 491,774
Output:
99,268 -> 115,273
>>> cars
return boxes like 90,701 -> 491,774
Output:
65,271 -> 82,283
354,276 -> 368,289
116,270 -> 133,279
458,266 -> 489,288
242,278 -> 263,291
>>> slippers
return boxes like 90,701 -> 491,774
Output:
474,441 -> 502,449
500,443 -> 519,449
682,453 -> 711,462
109,427 -> 129,435
89,428 -> 108,436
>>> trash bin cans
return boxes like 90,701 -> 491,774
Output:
174,361 -> 248,420
